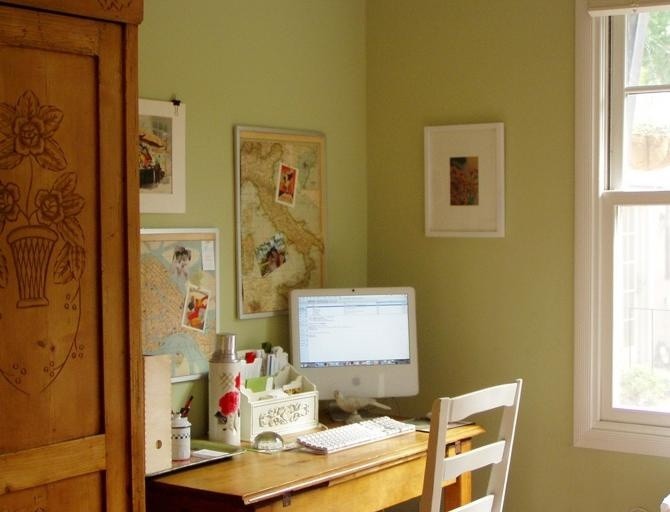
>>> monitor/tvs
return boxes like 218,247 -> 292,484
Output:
289,286 -> 419,422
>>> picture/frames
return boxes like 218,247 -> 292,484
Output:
140,228 -> 220,383
423,122 -> 506,239
139,97 -> 186,215
232,125 -> 327,319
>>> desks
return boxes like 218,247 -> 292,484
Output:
146,416 -> 486,512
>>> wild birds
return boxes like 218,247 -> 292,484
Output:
331,389 -> 393,415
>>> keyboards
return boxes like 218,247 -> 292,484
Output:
296,415 -> 416,454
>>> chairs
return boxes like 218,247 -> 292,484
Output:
420,378 -> 523,512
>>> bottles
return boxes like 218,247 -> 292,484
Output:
172,412 -> 192,461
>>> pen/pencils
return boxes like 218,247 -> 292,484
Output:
179,395 -> 194,416
